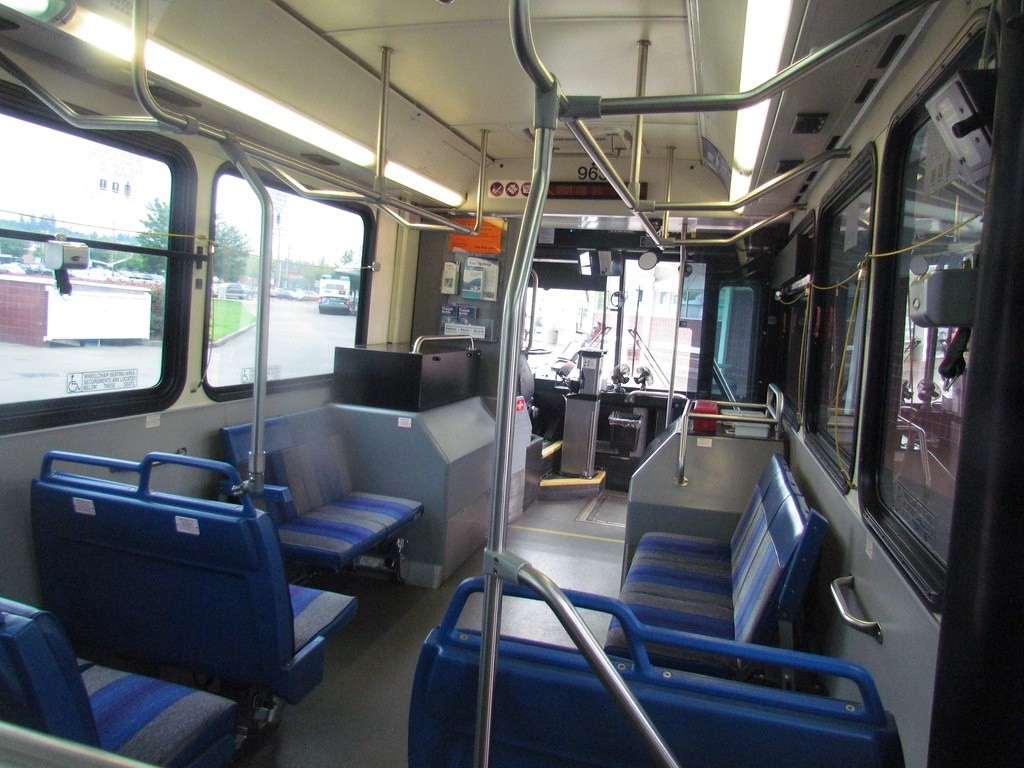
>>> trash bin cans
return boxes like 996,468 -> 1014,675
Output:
547,330 -> 558,344
609,410 -> 644,452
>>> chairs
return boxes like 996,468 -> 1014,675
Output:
518,352 -> 541,418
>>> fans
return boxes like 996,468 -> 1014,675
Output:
612,364 -> 630,391
635,366 -> 653,391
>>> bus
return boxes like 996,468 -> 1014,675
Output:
319,275 -> 350,314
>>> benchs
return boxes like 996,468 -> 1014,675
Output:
1,408 -> 904,768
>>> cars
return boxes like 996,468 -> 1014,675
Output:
0,254 -> 164,280
213,282 -> 318,302
